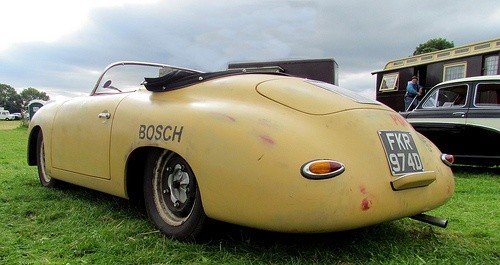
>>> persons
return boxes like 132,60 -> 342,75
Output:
381,80 -> 388,89
404,76 -> 423,112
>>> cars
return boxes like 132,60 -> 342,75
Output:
399,75 -> 500,166
1,107 -> 23,122
26,61 -> 455,241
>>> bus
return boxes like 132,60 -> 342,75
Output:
370,40 -> 500,116
